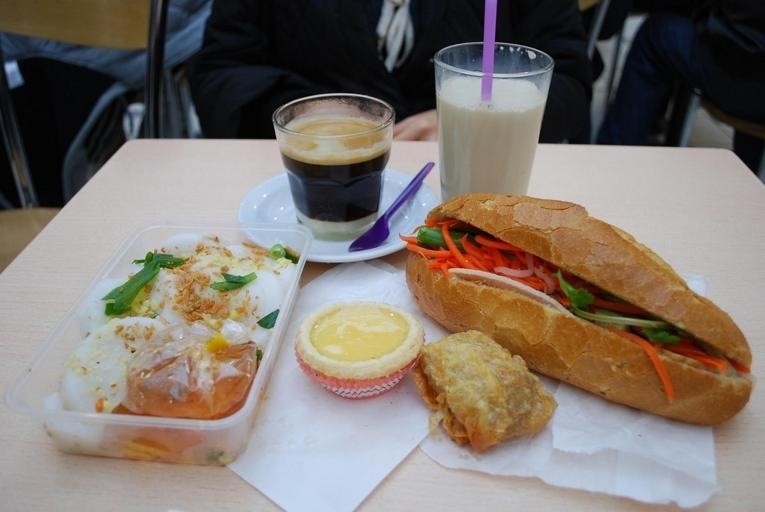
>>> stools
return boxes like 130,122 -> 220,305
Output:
655,87 -> 763,173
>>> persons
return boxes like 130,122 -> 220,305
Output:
595,0 -> 765,178
184,0 -> 604,144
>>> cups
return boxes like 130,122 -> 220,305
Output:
431,42 -> 555,204
271,93 -> 393,239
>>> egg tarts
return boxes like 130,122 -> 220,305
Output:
293,299 -> 425,381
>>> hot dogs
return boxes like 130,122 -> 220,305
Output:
398,191 -> 754,426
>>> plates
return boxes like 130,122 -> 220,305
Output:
237,165 -> 437,264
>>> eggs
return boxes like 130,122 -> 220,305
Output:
40,232 -> 294,419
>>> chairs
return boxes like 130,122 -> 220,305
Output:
0,0 -> 168,271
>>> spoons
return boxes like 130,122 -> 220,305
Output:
349,162 -> 435,251
